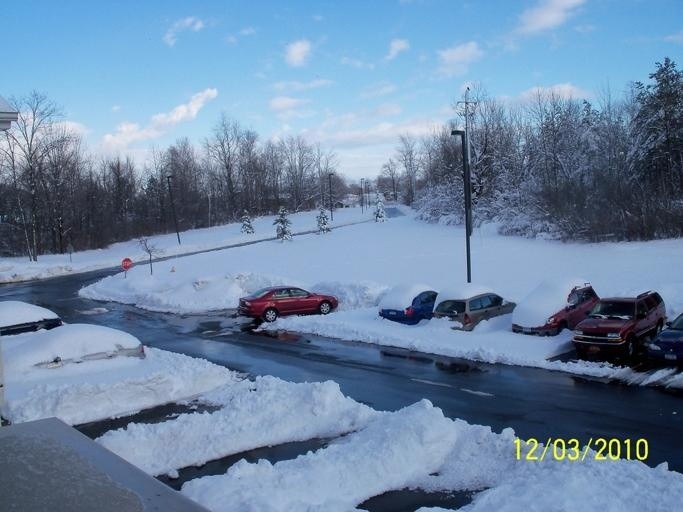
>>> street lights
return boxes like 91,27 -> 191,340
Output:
165,173 -> 181,245
451,126 -> 474,283
328,171 -> 372,222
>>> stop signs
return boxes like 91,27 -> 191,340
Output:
120,257 -> 132,271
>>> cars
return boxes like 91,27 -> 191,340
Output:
0,300 -> 148,369
237,285 -> 338,323
